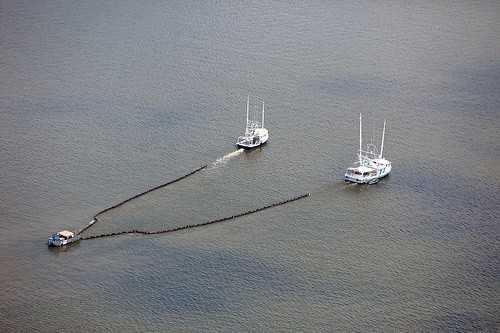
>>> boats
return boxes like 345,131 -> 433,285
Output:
344,113 -> 392,184
369,178 -> 379,186
236,95 -> 269,150
47,228 -> 80,247
